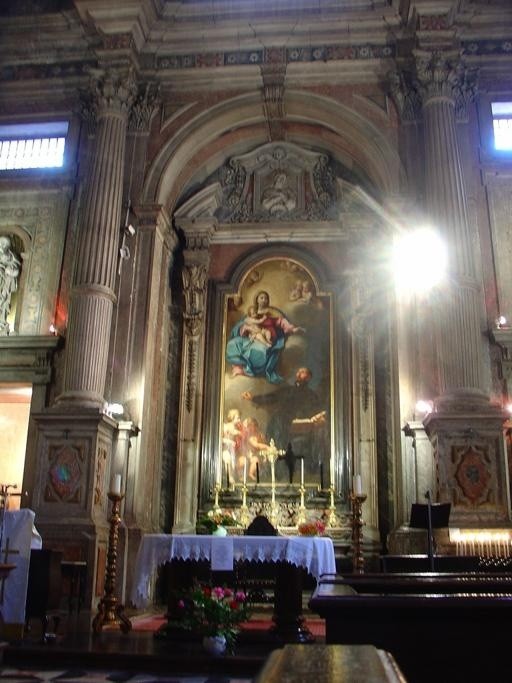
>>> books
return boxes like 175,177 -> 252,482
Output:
409,501 -> 450,529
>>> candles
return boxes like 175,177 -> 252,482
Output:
353,473 -> 363,495
110,473 -> 122,493
300,457 -> 304,484
271,461 -> 275,488
244,456 -> 247,485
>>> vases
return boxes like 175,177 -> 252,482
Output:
203,635 -> 227,655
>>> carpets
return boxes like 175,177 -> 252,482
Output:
104,611 -> 327,644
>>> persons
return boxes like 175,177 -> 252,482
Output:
262,171 -> 296,213
220,260 -> 329,484
1,234 -> 22,338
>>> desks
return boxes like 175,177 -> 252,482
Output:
141,532 -> 333,643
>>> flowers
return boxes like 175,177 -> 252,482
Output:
159,575 -> 254,656
